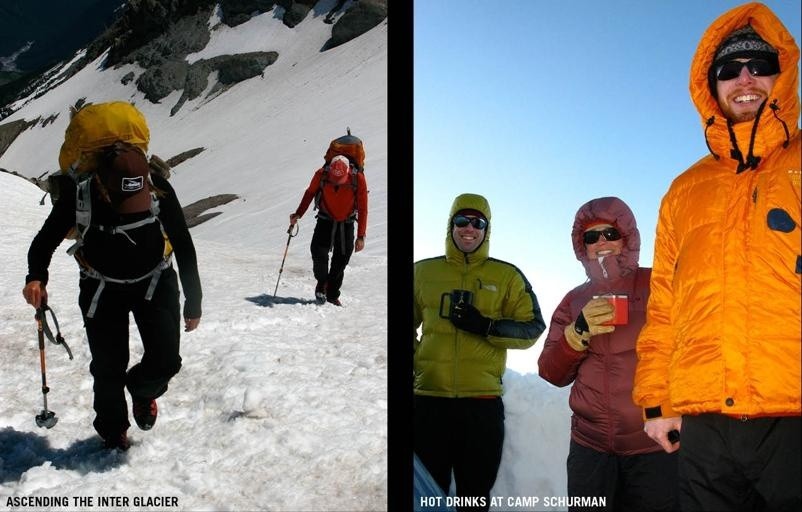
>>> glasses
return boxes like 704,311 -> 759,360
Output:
584,228 -> 621,244
716,58 -> 780,81
455,215 -> 487,229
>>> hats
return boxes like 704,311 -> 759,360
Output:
714,26 -> 778,65
331,155 -> 349,184
102,139 -> 151,213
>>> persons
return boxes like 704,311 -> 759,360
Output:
632,2 -> 802,510
23,148 -> 202,450
290,153 -> 368,305
413,194 -> 546,512
537,197 -> 678,511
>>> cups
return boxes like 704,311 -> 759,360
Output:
438,288 -> 474,320
594,295 -> 629,326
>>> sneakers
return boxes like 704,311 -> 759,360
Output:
315,281 -> 328,299
105,429 -> 127,450
327,298 -> 340,305
133,399 -> 157,430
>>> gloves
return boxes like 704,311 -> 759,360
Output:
451,303 -> 491,336
564,298 -> 616,351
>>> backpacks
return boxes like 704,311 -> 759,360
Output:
320,135 -> 365,192
47,99 -> 150,206
